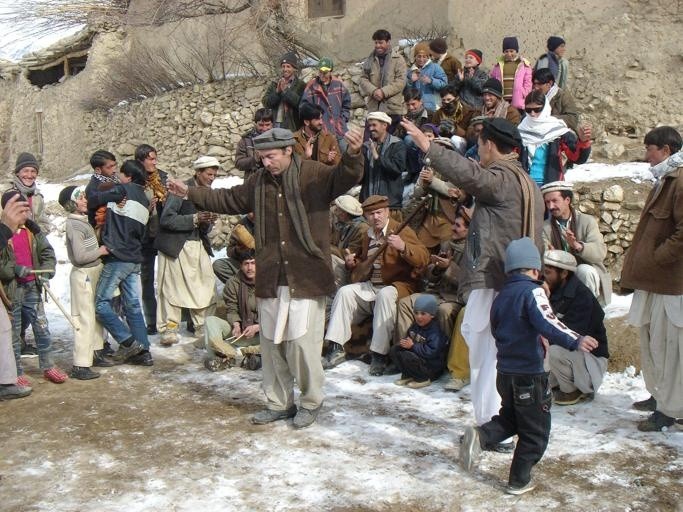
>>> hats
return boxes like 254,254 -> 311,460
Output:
548,36 -> 565,51
541,180 -> 574,195
252,128 -> 296,150
335,194 -> 390,217
504,236 -> 542,272
317,57 -> 333,72
59,184 -> 87,213
414,37 -> 447,59
470,115 -> 522,147
413,294 -> 437,316
503,37 -> 519,53
480,78 -> 502,99
193,155 -> 220,170
544,249 -> 577,272
280,51 -> 298,70
465,49 -> 482,65
366,111 -> 392,125
15,152 -> 39,174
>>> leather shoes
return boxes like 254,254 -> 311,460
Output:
147,324 -> 157,334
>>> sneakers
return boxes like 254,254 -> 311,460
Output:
321,348 -> 347,370
44,368 -> 68,384
637,411 -> 683,432
20,344 -> 39,359
634,396 -> 656,411
240,354 -> 262,370
459,425 -> 541,495
250,400 -> 323,427
369,354 -> 431,388
70,366 -> 100,381
205,356 -> 236,372
444,377 -> 470,391
0,375 -> 33,399
93,338 -> 154,366
160,323 -> 180,344
555,389 -> 591,405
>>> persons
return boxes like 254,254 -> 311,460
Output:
618,125 -> 682,434
2,144 -> 260,400
235,32 -> 611,406
458,237 -> 599,497
395,114 -> 545,457
162,121 -> 364,430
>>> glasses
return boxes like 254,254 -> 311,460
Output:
525,106 -> 544,114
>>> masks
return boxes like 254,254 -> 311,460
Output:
441,99 -> 457,111
